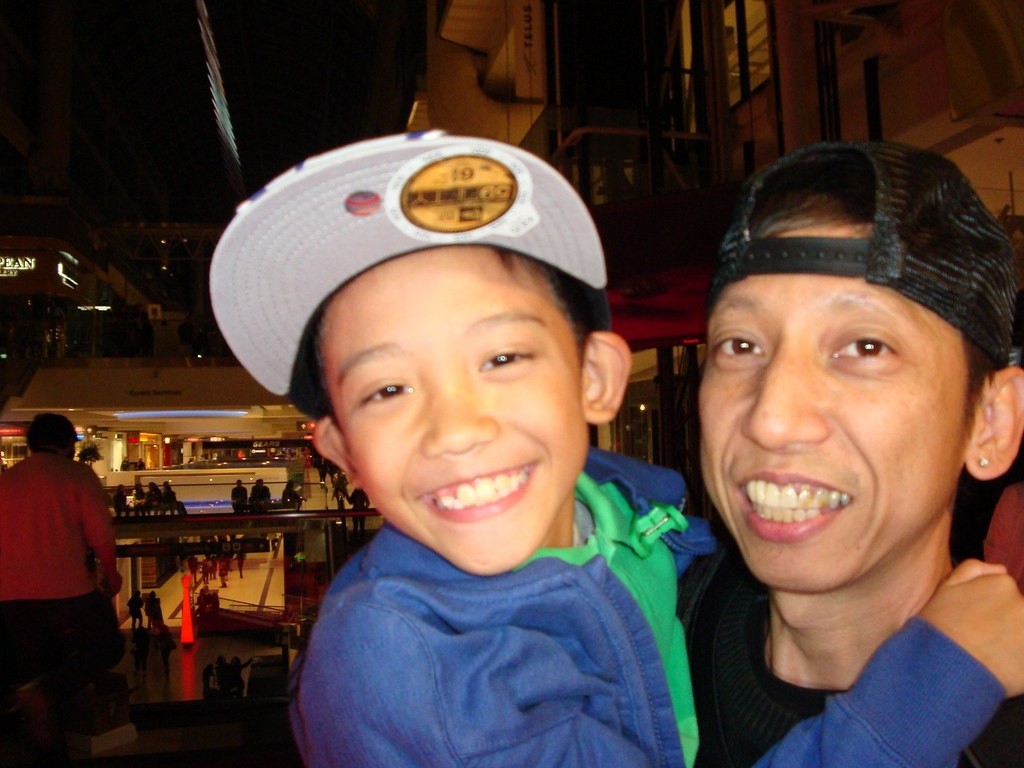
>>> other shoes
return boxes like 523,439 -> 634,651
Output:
14,682 -> 55,749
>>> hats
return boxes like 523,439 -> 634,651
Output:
26,413 -> 81,442
707,138 -> 1019,368
209,129 -> 607,422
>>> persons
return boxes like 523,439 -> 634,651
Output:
0,413 -> 124,744
231,461 -> 370,534
200,654 -> 253,699
669,136 -> 1024,768
210,131 -> 1024,768
108,457 -> 183,517
187,550 -> 246,624
126,590 -> 177,678
